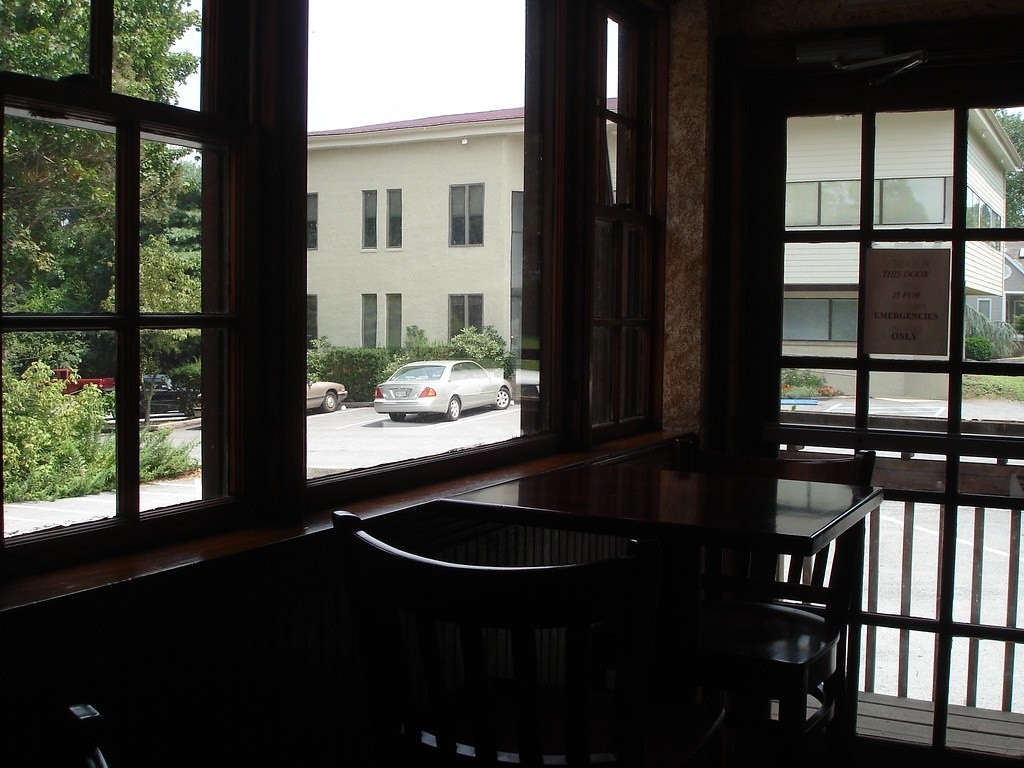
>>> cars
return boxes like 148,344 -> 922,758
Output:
374,360 -> 513,421
306,379 -> 348,413
138,373 -> 192,418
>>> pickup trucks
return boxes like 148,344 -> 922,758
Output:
47,369 -> 116,404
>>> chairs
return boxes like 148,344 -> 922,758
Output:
571,433 -> 878,768
330,508 -> 730,768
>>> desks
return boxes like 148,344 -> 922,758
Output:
437,465 -> 884,768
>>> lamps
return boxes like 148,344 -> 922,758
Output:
461,137 -> 468,145
